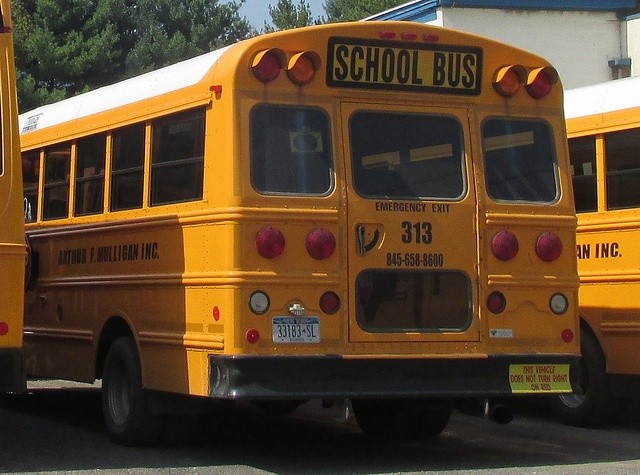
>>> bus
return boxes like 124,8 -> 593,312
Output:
546,105 -> 640,420
0,0 -> 28,397
18,20 -> 582,458
